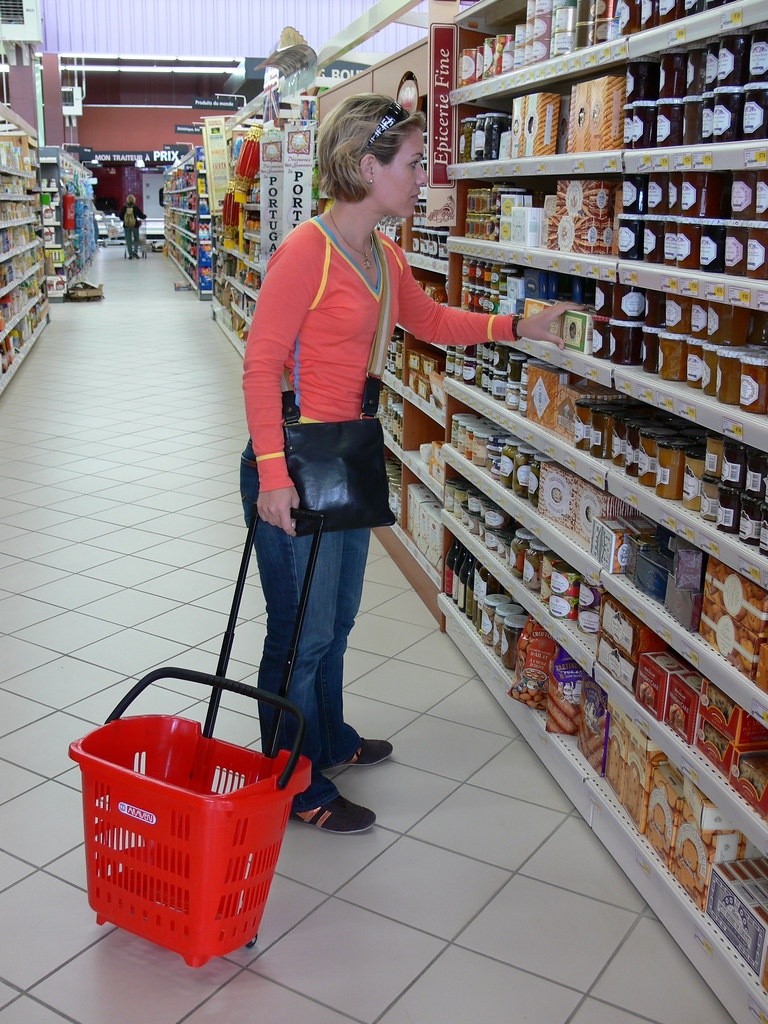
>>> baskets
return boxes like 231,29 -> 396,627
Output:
69,716 -> 311,967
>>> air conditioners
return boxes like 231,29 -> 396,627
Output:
0,0 -> 43,46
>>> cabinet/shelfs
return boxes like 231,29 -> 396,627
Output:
160,0 -> 768,1024
0,127 -> 98,395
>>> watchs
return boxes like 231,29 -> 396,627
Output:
512,314 -> 525,341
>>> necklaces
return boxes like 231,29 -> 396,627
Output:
328,207 -> 374,273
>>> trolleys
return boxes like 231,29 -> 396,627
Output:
122,218 -> 148,260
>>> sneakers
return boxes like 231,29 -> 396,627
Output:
288,795 -> 377,833
320,738 -> 393,772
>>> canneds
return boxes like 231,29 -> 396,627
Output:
378,0 -> 768,669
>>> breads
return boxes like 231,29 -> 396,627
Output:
512,685 -> 607,775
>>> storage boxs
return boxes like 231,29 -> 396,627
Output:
174,281 -> 191,290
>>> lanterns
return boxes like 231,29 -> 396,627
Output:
233,123 -> 264,205
222,179 -> 240,251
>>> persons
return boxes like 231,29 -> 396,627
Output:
239,94 -> 589,838
120,195 -> 147,259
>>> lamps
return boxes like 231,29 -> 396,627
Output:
135,158 -> 145,168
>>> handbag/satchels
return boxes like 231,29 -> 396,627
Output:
283,419 -> 397,537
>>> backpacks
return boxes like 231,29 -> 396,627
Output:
125,204 -> 135,228
